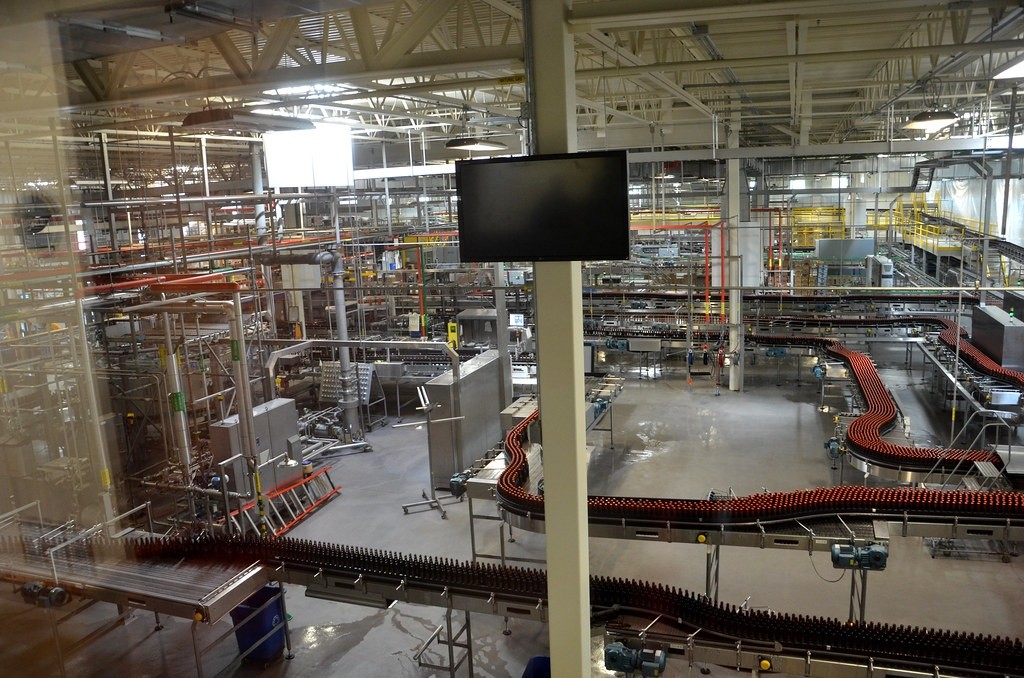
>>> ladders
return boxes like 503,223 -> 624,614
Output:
216,465 -> 344,541
220,470 -> 310,539
914,409 -> 1012,493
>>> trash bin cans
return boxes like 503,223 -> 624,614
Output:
229,584 -> 287,670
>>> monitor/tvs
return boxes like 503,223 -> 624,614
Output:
455,148 -> 631,263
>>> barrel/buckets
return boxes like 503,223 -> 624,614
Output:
229,586 -> 286,669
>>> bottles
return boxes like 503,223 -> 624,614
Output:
497,409 -> 544,515
588,485 -> 1024,678
581,289 -> 1024,475
0,527 -> 547,607
